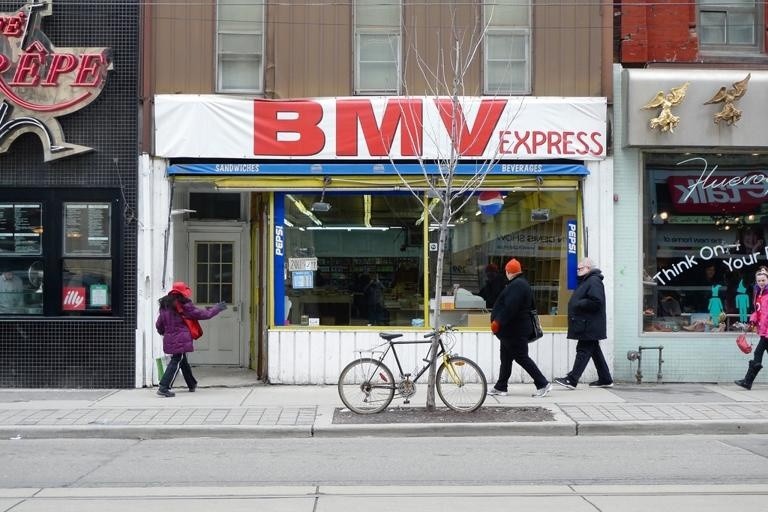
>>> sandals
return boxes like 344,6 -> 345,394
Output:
645,308 -> 653,316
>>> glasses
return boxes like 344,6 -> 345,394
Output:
577,266 -> 585,271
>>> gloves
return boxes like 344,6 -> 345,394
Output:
491,320 -> 500,334
217,301 -> 228,311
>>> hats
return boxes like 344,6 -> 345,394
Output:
485,264 -> 498,273
505,258 -> 521,273
169,282 -> 193,298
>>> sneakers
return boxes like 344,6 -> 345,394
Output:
682,320 -> 705,331
532,382 -> 552,398
188,381 -> 198,392
157,385 -> 176,397
487,385 -> 508,396
554,377 -> 577,390
588,380 -> 614,388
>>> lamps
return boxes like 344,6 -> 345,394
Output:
528,174 -> 552,222
648,203 -> 762,238
309,174 -> 334,212
424,176 -> 448,199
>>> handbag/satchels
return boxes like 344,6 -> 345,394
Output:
528,309 -> 544,343
736,327 -> 753,355
183,318 -> 203,340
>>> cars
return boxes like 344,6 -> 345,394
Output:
0,266 -> 110,315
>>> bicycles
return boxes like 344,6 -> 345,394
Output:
336,319 -> 488,417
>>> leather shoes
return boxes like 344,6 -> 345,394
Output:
644,322 -> 681,332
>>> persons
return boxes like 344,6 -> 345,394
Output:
690,262 -> 716,307
728,225 -> 766,255
712,88 -> 745,128
485,257 -> 553,397
472,262 -> 507,308
734,264 -> 768,390
156,281 -> 228,397
359,272 -> 390,327
552,256 -> 614,391
0,267 -> 25,307
647,94 -> 682,135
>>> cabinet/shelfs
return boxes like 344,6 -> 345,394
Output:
281,243 -> 496,331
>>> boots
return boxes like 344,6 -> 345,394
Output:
734,359 -> 764,390
